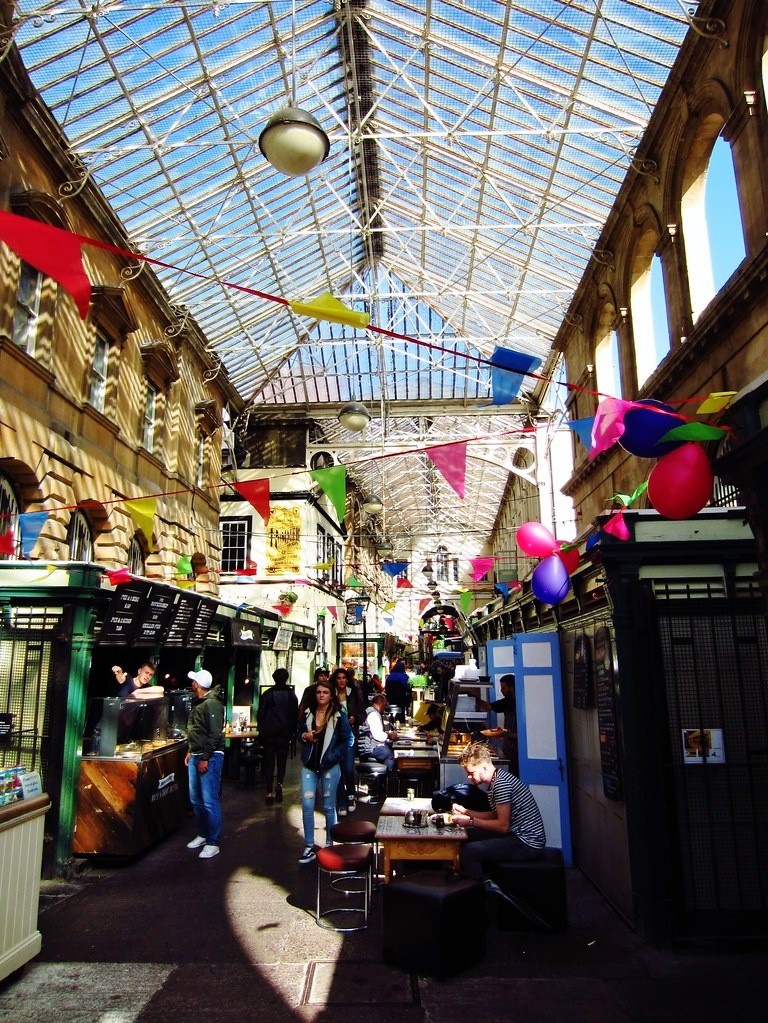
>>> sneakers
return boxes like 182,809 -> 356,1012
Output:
348,795 -> 356,811
187,836 -> 206,848
198,845 -> 220,858
299,845 -> 316,863
338,805 -> 347,816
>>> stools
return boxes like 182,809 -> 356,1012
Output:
314,742 -> 392,937
496,850 -> 568,933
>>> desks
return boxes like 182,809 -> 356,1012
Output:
54,736 -> 189,864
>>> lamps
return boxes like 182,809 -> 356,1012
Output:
363,437 -> 444,614
257,0 -> 332,175
337,326 -> 372,433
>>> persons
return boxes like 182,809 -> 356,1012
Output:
452,745 -> 546,896
256,668 -> 298,806
92,662 -> 154,752
477,673 -> 519,780
298,656 -> 461,815
295,681 -> 354,864
184,669 -> 225,859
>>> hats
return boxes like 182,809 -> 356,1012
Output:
188,670 -> 212,688
315,667 -> 330,675
425,704 -> 441,715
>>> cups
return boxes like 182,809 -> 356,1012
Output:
420,810 -> 428,825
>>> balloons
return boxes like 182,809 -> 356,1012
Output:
648,443 -> 714,519
543,540 -> 580,574
531,555 -> 569,605
517,521 -> 557,556
618,397 -> 690,458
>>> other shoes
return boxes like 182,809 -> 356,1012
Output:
265,797 -> 273,806
275,784 -> 283,803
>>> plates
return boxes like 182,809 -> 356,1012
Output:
459,678 -> 478,682
430,813 -> 453,825
397,741 -> 414,744
480,729 -> 508,736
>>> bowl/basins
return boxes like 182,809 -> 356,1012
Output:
478,676 -> 490,682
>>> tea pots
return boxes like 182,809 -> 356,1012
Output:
404,811 -> 419,824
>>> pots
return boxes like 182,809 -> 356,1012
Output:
451,733 -> 471,742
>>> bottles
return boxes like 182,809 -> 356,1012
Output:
407,788 -> 415,801
0,764 -> 26,794
225,721 -> 229,733
436,809 -> 444,829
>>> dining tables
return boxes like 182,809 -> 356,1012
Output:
224,721 -> 263,796
371,724 -> 472,931
